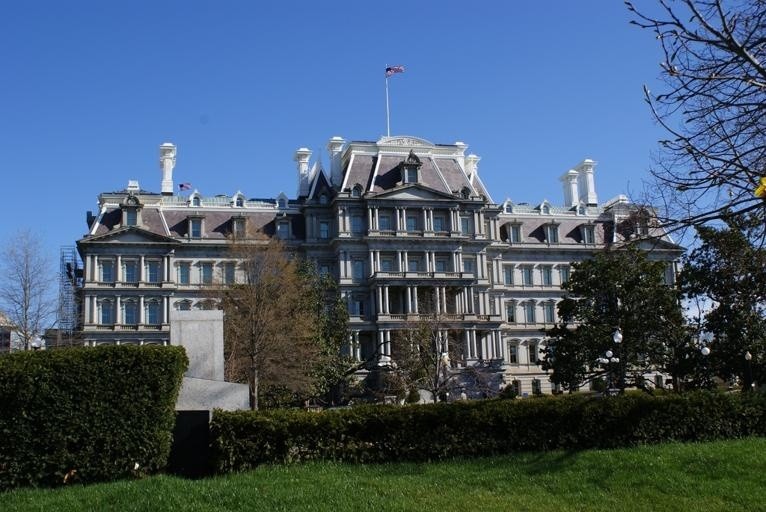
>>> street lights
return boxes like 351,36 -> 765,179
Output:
30,337 -> 42,351
601,350 -> 621,394
700,344 -> 712,388
743,349 -> 753,391
614,321 -> 627,394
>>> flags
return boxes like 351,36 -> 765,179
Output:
179,182 -> 193,192
385,65 -> 408,76
